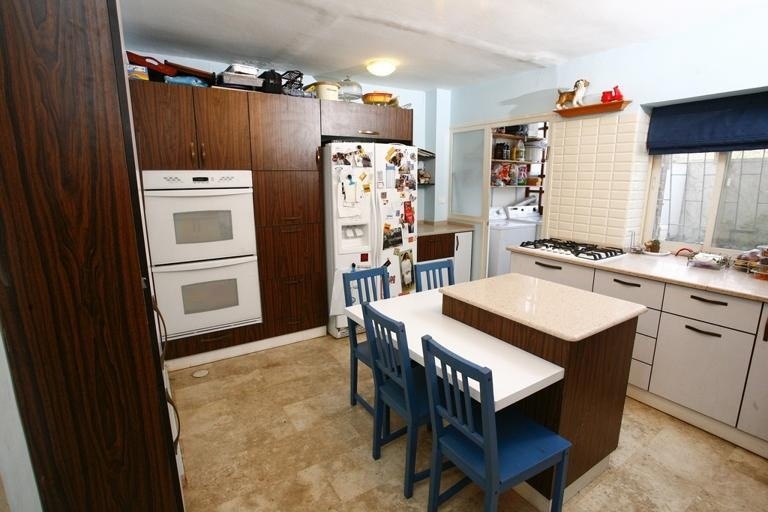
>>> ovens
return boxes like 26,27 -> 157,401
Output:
141,167 -> 267,343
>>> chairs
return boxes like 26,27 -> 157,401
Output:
412,257 -> 454,293
351,300 -> 457,498
421,333 -> 569,512
337,263 -> 401,420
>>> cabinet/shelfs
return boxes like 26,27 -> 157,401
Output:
243,89 -> 324,173
505,252 -> 594,293
419,155 -> 435,188
456,231 -> 473,285
733,299 -> 767,449
0,2 -> 186,510
252,170 -> 327,342
486,133 -> 546,190
315,98 -> 415,145
645,280 -> 764,430
586,268 -> 665,394
129,80 -> 251,171
417,233 -> 455,264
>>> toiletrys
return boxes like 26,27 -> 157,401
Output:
519,164 -> 528,184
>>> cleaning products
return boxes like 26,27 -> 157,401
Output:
510,164 -> 519,185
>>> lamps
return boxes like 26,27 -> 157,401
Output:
364,56 -> 398,78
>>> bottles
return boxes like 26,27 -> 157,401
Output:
502,143 -> 517,161
515,140 -> 526,162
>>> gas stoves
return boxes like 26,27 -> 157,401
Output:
520,236 -> 628,262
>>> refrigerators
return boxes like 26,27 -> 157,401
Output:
323,140 -> 418,340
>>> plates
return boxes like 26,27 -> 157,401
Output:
644,249 -> 671,258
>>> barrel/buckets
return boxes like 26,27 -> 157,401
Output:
526,144 -> 545,163
315,80 -> 342,102
517,139 -> 525,161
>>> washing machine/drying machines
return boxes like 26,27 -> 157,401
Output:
488,206 -> 537,278
505,204 -> 543,241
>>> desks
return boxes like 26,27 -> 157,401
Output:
340,282 -> 566,415
